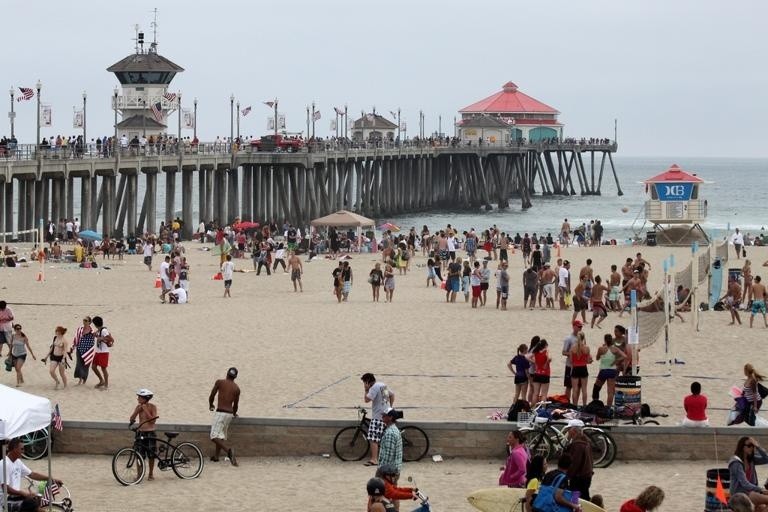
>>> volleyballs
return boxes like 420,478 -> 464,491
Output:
620,206 -> 629,214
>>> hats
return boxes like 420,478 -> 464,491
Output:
228,368 -> 238,376
565,419 -> 584,429
573,321 -> 583,326
380,407 -> 396,416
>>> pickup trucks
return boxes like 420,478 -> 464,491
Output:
246,134 -> 304,154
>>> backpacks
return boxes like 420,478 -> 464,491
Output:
402,250 -> 409,259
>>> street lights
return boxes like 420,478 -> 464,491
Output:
6,86 -> 16,136
141,94 -> 147,137
192,96 -> 199,138
176,89 -> 182,140
235,100 -> 240,138
35,79 -> 43,150
229,93 -> 235,142
273,89 -> 459,139
112,85 -> 119,138
81,91 -> 89,153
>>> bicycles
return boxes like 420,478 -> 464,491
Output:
332,408 -> 429,464
0,385 -> 49,460
508,415 -> 616,470
621,403 -> 660,426
2,481 -> 74,512
111,416 -> 204,487
516,399 -> 607,465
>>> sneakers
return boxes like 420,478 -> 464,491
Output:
228,448 -> 235,464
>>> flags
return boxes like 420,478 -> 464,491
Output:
40,479 -> 59,507
261,101 -> 274,109
151,102 -> 163,121
241,106 -> 252,116
311,111 -> 322,122
333,107 -> 345,116
162,92 -> 178,102
50,403 -> 62,434
15,95 -> 31,103
18,87 -> 35,100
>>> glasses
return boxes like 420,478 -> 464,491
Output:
745,443 -> 754,448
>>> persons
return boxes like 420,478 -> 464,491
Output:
5,323 -> 37,388
521,218 -> 652,330
376,407 -> 403,512
207,367 -> 242,466
128,388 -> 158,481
367,464 -> 419,512
555,418 -> 594,502
619,485 -> 666,512
728,435 -> 768,512
366,476 -> 397,512
42,325 -> 69,392
91,316 -> 114,388
525,454 -> 549,512
683,381 -> 710,428
1,215 -> 525,311
0,299 -> 14,359
728,492 -> 756,512
67,315 -> 96,386
0,131 -> 612,161
360,372 -> 395,466
676,227 -> 768,331
0,437 -> 64,512
498,429 -> 531,489
739,363 -> 763,426
532,453 -> 583,512
507,331 -> 633,414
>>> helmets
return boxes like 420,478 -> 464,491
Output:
368,464 -> 400,496
136,389 -> 154,396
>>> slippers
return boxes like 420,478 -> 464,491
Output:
363,462 -> 377,466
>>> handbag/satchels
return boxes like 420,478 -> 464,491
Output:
368,275 -> 373,283
758,383 -> 768,398
532,473 -> 572,512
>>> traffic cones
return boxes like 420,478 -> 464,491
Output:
441,276 -> 447,290
154,271 -> 164,289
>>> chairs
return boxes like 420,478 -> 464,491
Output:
242,233 -> 378,254
0,245 -> 102,267
